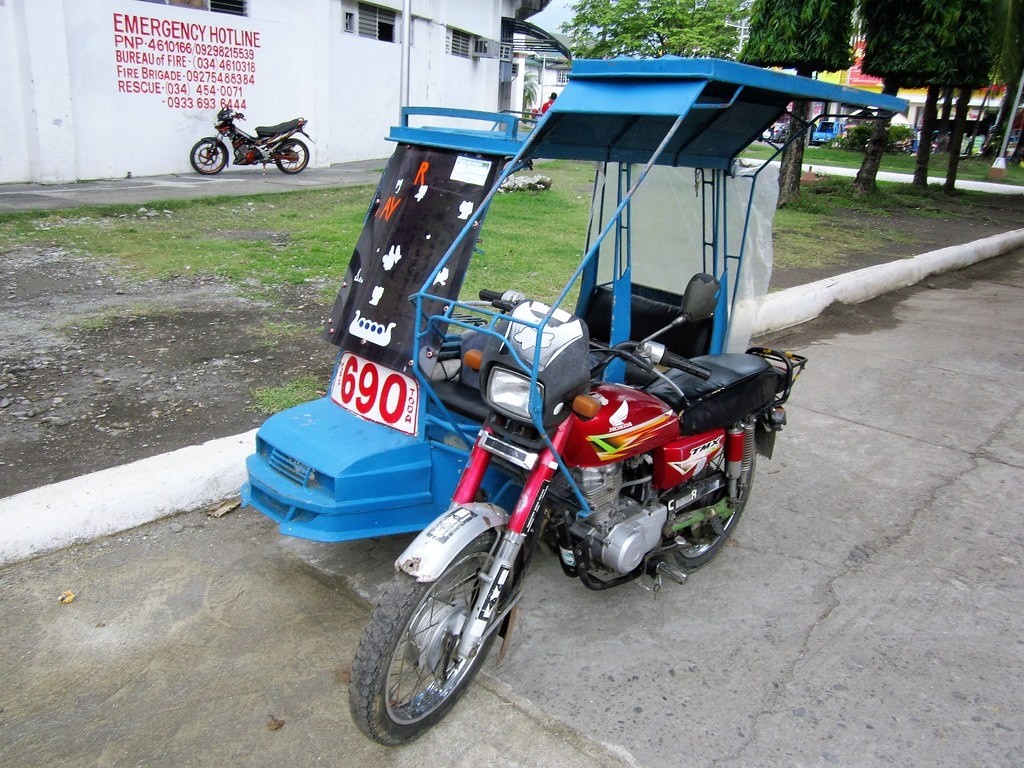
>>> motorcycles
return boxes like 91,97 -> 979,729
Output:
240,54 -> 910,750
190,104 -> 317,176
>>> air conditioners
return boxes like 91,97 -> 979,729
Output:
500,44 -> 514,63
471,37 -> 500,59
512,63 -> 520,77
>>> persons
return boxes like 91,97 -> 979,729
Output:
542,92 -> 557,114
899,134 -> 915,156
963,134 -> 973,155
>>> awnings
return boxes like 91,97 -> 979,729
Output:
501,16 -> 572,61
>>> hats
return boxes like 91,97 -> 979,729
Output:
550,92 -> 557,100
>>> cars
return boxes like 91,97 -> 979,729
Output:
764,122 -> 788,143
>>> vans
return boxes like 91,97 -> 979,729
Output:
811,120 -> 848,147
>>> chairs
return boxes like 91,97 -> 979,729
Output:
584,284 -> 716,377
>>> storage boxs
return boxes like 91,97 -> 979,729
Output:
962,135 -> 986,147
